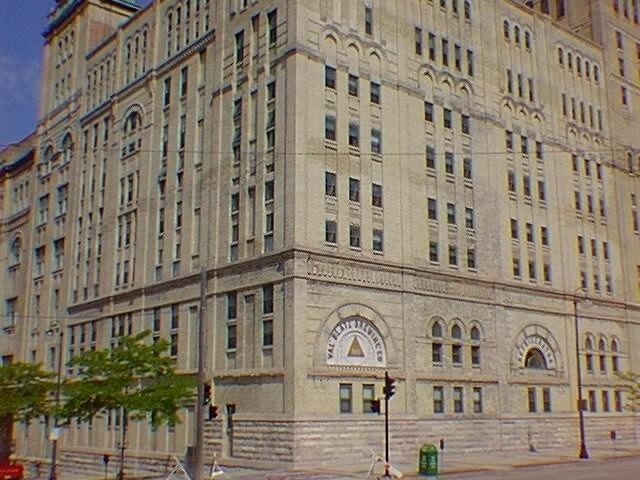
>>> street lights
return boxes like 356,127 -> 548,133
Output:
45,319 -> 66,479
572,286 -> 596,459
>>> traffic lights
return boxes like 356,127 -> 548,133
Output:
386,378 -> 395,400
210,406 -> 217,419
203,382 -> 211,405
